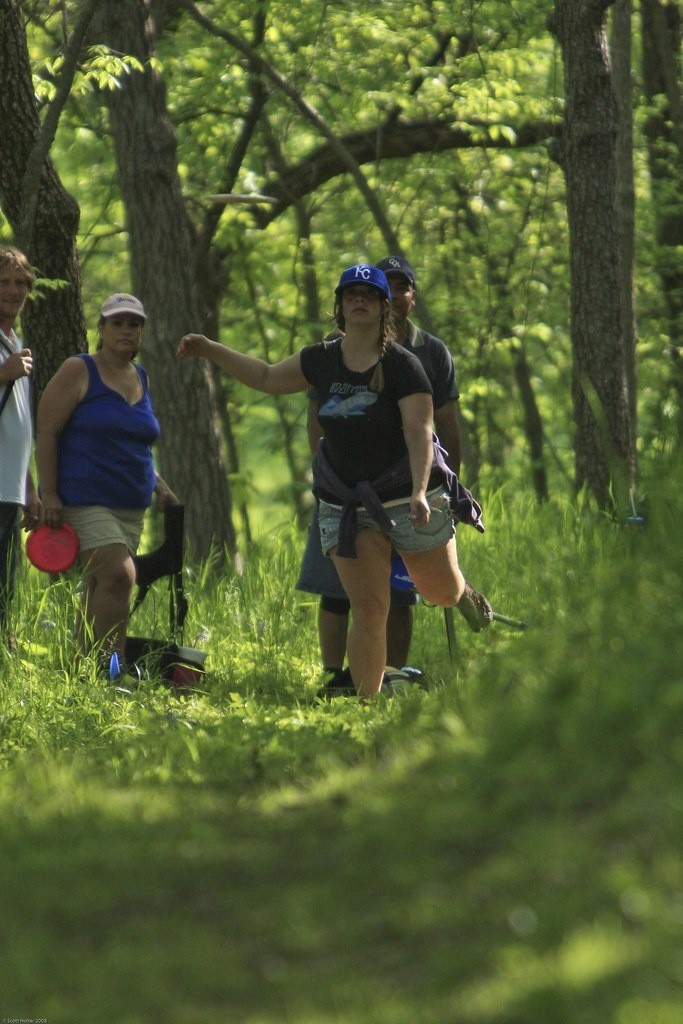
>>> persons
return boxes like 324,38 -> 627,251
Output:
175,265 -> 493,707
294,257 -> 460,678
35,294 -> 179,696
0,246 -> 44,653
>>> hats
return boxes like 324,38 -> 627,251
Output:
375,256 -> 416,286
100,293 -> 148,320
335,264 -> 392,301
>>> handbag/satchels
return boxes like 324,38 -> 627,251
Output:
125,637 -> 211,695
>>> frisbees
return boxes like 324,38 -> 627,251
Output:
25,520 -> 79,574
